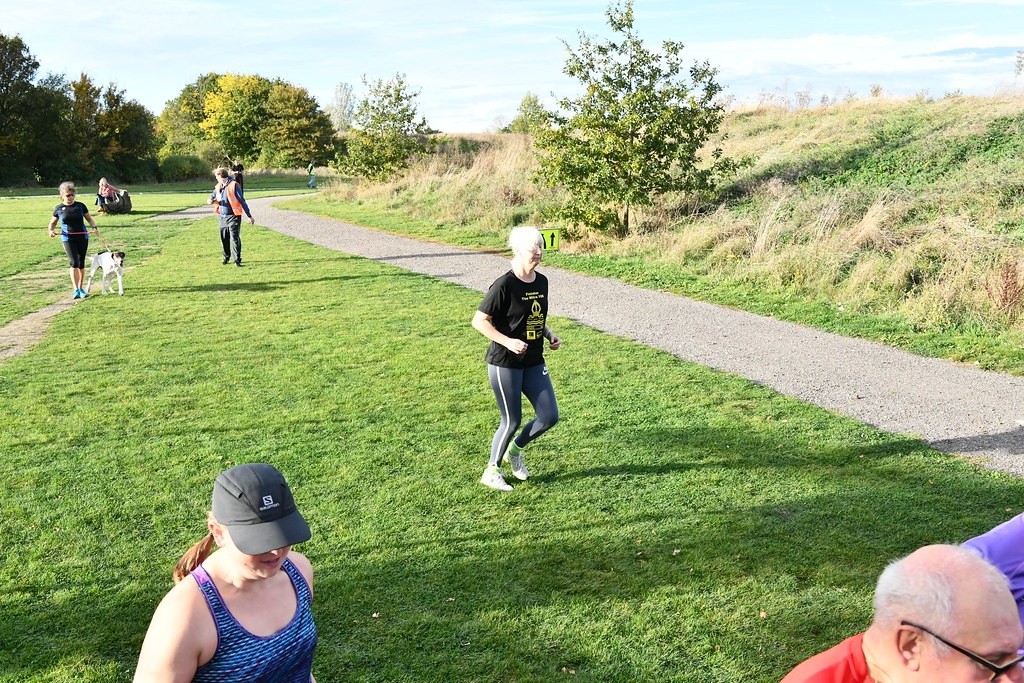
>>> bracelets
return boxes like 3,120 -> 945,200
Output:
544,329 -> 552,337
92,226 -> 97,229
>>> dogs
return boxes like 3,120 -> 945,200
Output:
87,251 -> 125,296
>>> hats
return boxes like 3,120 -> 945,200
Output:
213,463 -> 311,555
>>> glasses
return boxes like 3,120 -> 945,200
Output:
901,620 -> 1024,683
66,194 -> 74,197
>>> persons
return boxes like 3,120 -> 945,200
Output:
48,181 -> 98,299
306,160 -> 317,188
777,513 -> 1024,683
207,168 -> 255,268
96,178 -> 128,212
470,227 -> 563,491
131,463 -> 318,683
232,160 -> 244,199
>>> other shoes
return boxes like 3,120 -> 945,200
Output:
235,262 -> 243,268
223,260 -> 229,264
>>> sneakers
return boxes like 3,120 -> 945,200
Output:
480,468 -> 513,491
80,288 -> 88,298
503,449 -> 530,480
73,290 -> 80,299
